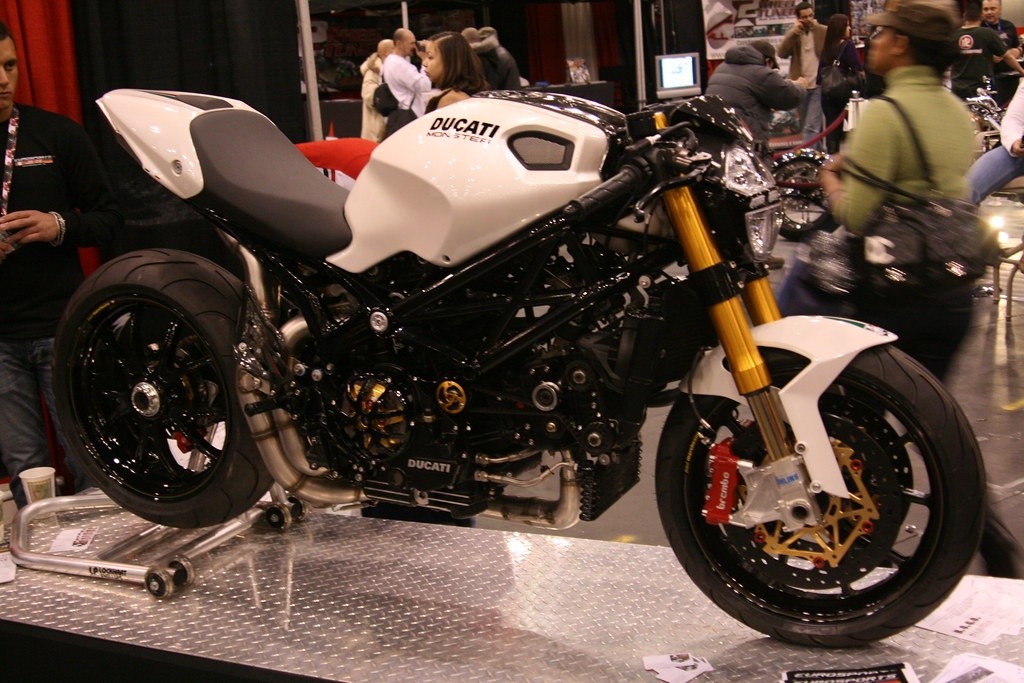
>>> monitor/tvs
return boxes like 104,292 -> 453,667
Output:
654,53 -> 702,100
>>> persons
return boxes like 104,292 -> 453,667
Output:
815,14 -> 861,155
945,0 -> 1024,102
777,2 -> 828,149
775,0 -> 973,382
705,40 -> 808,141
1,22 -> 123,510
966,77 -> 1024,206
360,27 -> 522,142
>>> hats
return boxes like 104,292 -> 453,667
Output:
865,1 -> 957,43
477,26 -> 496,39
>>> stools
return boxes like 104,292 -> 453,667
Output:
990,176 -> 1024,322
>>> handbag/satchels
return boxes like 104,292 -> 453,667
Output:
372,83 -> 398,118
843,94 -> 989,294
820,40 -> 868,105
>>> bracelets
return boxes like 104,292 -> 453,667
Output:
48,211 -> 66,246
818,168 -> 841,177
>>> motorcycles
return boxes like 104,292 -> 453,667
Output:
42,82 -> 994,654
770,73 -> 1008,250
